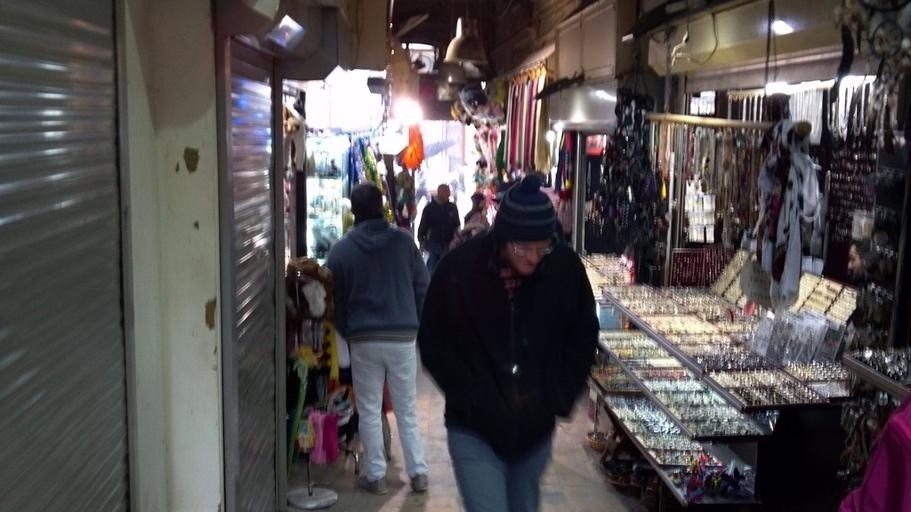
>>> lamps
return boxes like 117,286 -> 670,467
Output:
444,2 -> 488,65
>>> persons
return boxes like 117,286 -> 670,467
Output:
847,238 -> 885,288
839,395 -> 911,512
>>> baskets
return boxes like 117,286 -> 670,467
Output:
607,470 -> 641,496
585,431 -> 618,451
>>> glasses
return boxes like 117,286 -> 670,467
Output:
510,241 -> 556,257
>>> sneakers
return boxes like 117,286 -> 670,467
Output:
357,474 -> 389,496
410,475 -> 429,492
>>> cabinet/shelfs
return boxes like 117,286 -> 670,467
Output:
588,286 -> 840,511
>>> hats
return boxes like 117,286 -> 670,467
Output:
495,174 -> 559,239
